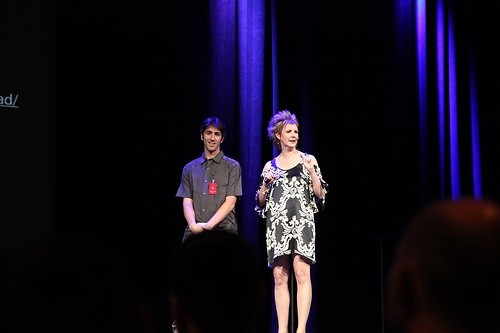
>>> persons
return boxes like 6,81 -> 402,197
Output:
388,200 -> 500,333
255,110 -> 328,333
176,116 -> 243,243
